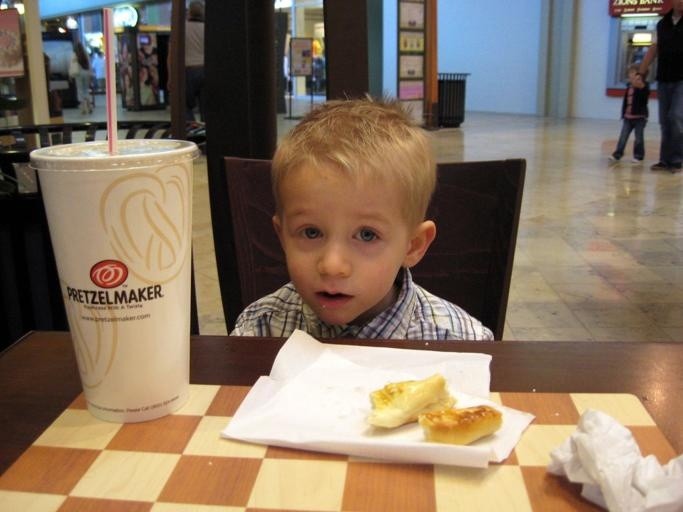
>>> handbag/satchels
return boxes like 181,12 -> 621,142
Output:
68,64 -> 80,78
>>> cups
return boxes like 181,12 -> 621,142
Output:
25,136 -> 203,424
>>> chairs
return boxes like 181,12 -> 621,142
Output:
218,155 -> 529,338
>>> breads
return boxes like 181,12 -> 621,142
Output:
370,374 -> 455,427
419,405 -> 502,444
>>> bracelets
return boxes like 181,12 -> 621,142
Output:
634,72 -> 644,78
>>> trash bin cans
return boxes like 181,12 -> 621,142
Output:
438,72 -> 471,128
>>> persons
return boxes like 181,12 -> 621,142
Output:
69,43 -> 93,116
607,63 -> 652,164
225,93 -> 494,341
308,59 -> 326,94
632,1 -> 682,175
116,32 -> 160,110
292,49 -> 312,75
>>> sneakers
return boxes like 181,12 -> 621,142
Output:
608,155 -> 683,174
80,107 -> 92,114
